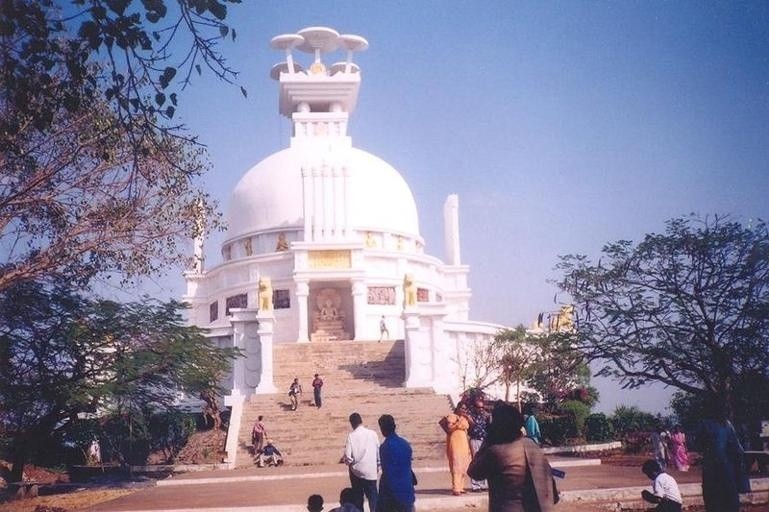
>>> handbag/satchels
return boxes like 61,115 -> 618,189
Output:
523,468 -> 560,511
665,446 -> 673,461
439,416 -> 451,434
729,432 -> 752,494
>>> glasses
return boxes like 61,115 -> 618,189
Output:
475,406 -> 485,409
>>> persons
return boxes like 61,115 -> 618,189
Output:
378,315 -> 390,344
275,232 -> 289,252
466,397 -> 492,492
465,401 -> 554,512
331,487 -> 362,511
289,377 -> 303,411
640,394 -> 769,511
255,438 -> 282,467
307,493 -> 324,511
250,416 -> 268,455
312,373 -> 323,409
521,402 -> 542,447
342,412 -> 379,511
320,299 -> 339,322
442,401 -> 475,496
375,413 -> 416,512
